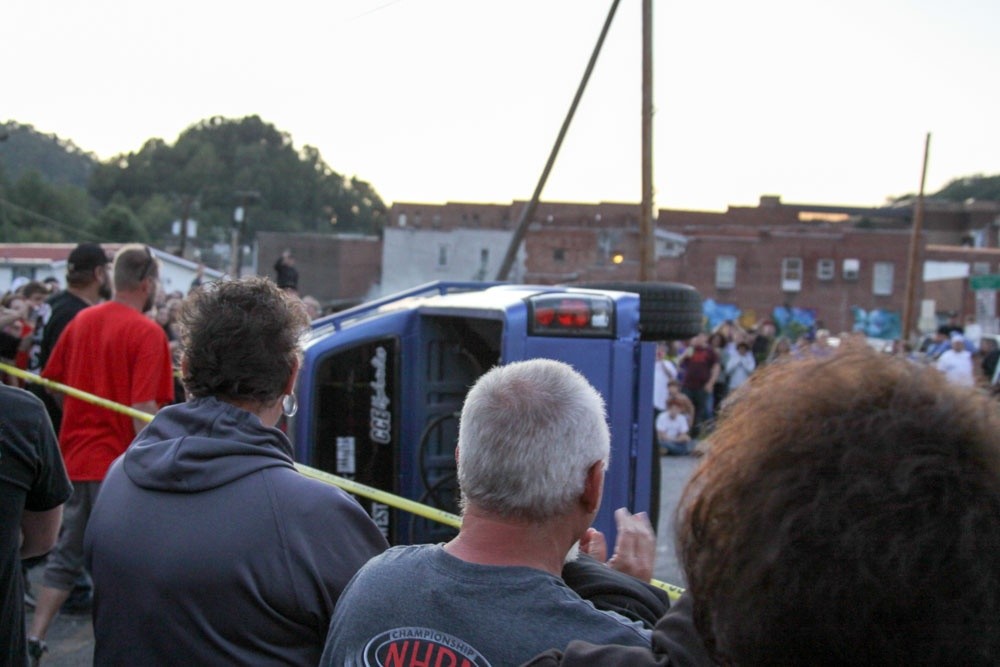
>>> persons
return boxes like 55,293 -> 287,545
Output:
22,243 -> 112,439
899,325 -> 1000,392
275,251 -> 297,289
26,244 -> 175,667
0,381 -> 75,667
317,359 -> 656,667
190,264 -> 203,286
81,276 -> 389,667
146,289 -> 183,353
0,277 -> 60,371
653,319 -> 875,461
523,338 -> 1000,667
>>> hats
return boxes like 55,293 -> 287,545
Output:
67,242 -> 112,273
9,277 -> 29,293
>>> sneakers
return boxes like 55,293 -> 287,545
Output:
27,637 -> 48,667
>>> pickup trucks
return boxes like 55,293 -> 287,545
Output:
283,274 -> 709,574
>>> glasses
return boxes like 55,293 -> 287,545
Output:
140,246 -> 156,280
